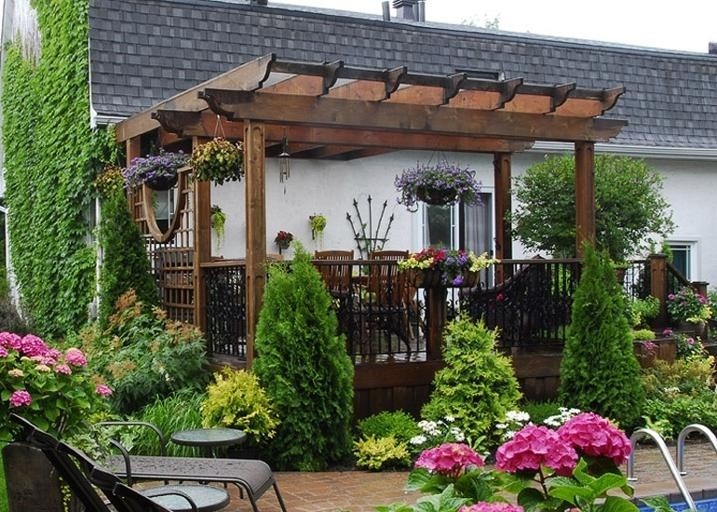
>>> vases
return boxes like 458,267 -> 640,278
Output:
279,242 -> 289,248
678,319 -> 705,339
2,442 -> 86,512
417,184 -> 457,206
205,165 -> 217,180
142,172 -> 178,191
411,268 -> 479,287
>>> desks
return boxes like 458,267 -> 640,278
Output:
171,428 -> 247,499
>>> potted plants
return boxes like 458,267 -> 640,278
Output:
309,215 -> 326,240
210,206 -> 226,250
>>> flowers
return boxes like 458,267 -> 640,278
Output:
394,159 -> 488,213
396,246 -> 501,274
666,287 -> 712,324
120,148 -> 190,196
192,137 -> 245,185
0,331 -> 112,446
274,230 -> 294,243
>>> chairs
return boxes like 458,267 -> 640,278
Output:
10,412 -> 232,512
94,421 -> 288,512
310,249 -> 426,357
457,254 -> 550,351
